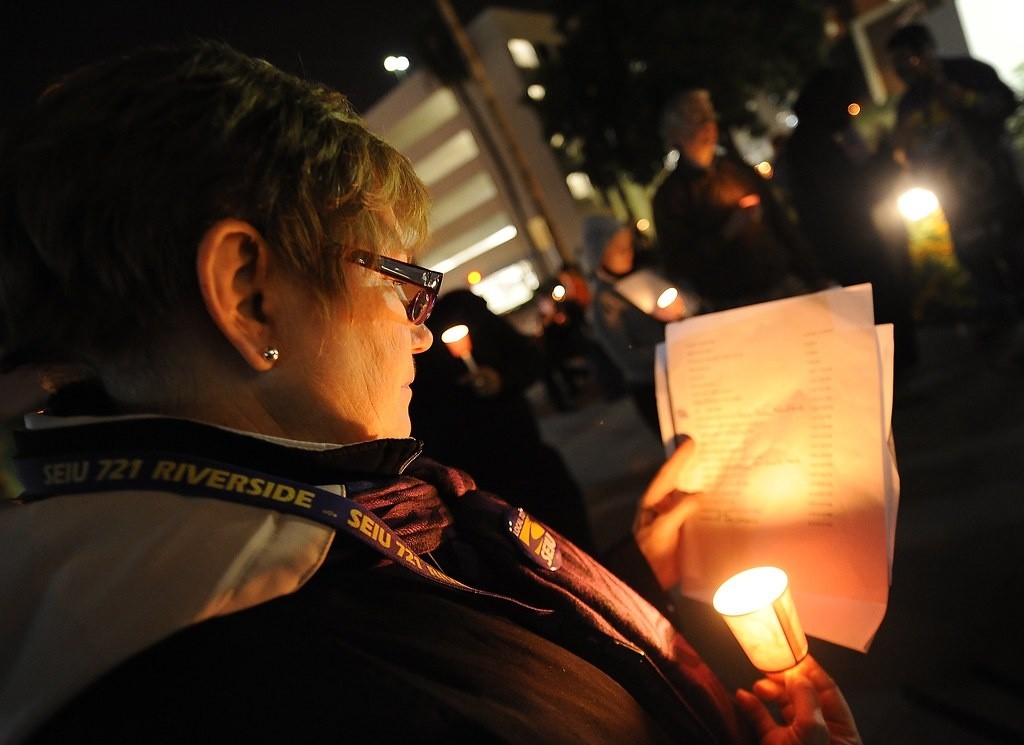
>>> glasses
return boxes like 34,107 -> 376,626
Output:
337,240 -> 443,325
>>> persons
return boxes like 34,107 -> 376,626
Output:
0,44 -> 863,745
409,21 -> 1024,561
